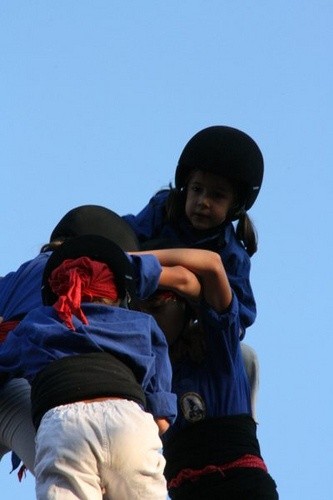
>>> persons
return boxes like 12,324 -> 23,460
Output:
120,247 -> 282,499
1,238 -> 181,500
119,124 -> 263,341
0,204 -> 200,480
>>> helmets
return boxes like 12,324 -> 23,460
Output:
176,125 -> 264,220
48,204 -> 140,254
42,234 -> 132,308
